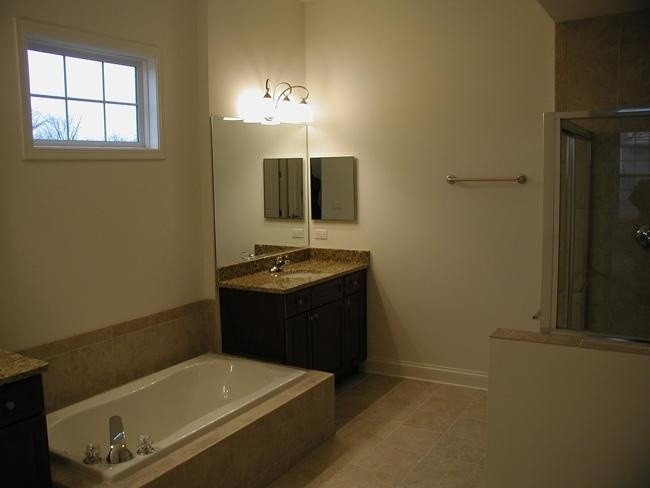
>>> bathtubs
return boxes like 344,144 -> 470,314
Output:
46,353 -> 307,484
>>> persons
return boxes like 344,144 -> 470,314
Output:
311,166 -> 321,220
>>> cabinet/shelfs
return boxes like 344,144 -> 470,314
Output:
220,271 -> 368,381
1,375 -> 51,488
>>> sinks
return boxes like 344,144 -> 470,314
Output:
274,270 -> 324,278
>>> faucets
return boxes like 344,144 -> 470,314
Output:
241,250 -> 254,261
106,416 -> 133,463
270,256 -> 290,272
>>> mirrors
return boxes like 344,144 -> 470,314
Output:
262,158 -> 303,220
209,115 -> 355,272
